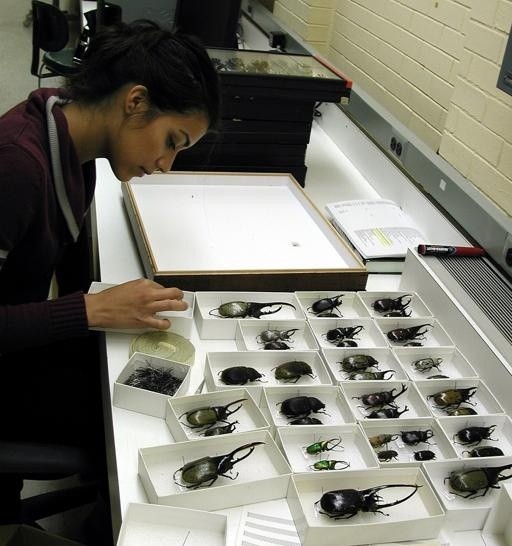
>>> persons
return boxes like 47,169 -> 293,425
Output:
0,17 -> 218,511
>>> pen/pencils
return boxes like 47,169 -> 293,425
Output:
418,243 -> 486,256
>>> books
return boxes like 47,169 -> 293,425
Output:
324,199 -> 428,262
326,217 -> 406,274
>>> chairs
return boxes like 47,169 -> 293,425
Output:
31,0 -> 83,89
0,439 -> 96,532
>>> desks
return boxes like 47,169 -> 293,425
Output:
88,120 -> 512,546
